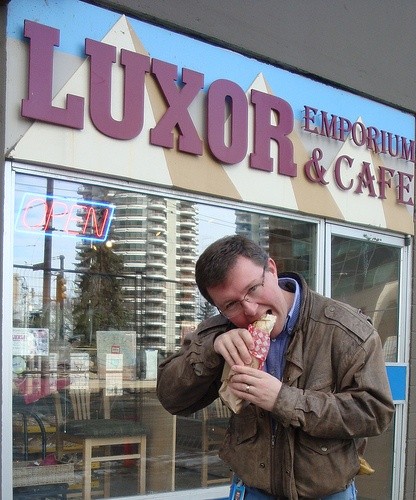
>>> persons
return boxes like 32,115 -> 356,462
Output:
157,235 -> 395,499
234,492 -> 241,500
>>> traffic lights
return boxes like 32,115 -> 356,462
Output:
55,275 -> 68,303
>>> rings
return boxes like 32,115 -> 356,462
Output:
245,385 -> 250,394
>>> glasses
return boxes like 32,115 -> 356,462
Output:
216,263 -> 266,319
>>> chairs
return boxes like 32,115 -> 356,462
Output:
176,399 -> 232,488
53,368 -> 147,500
13,408 -> 69,499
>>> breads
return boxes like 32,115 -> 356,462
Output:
252,313 -> 276,333
